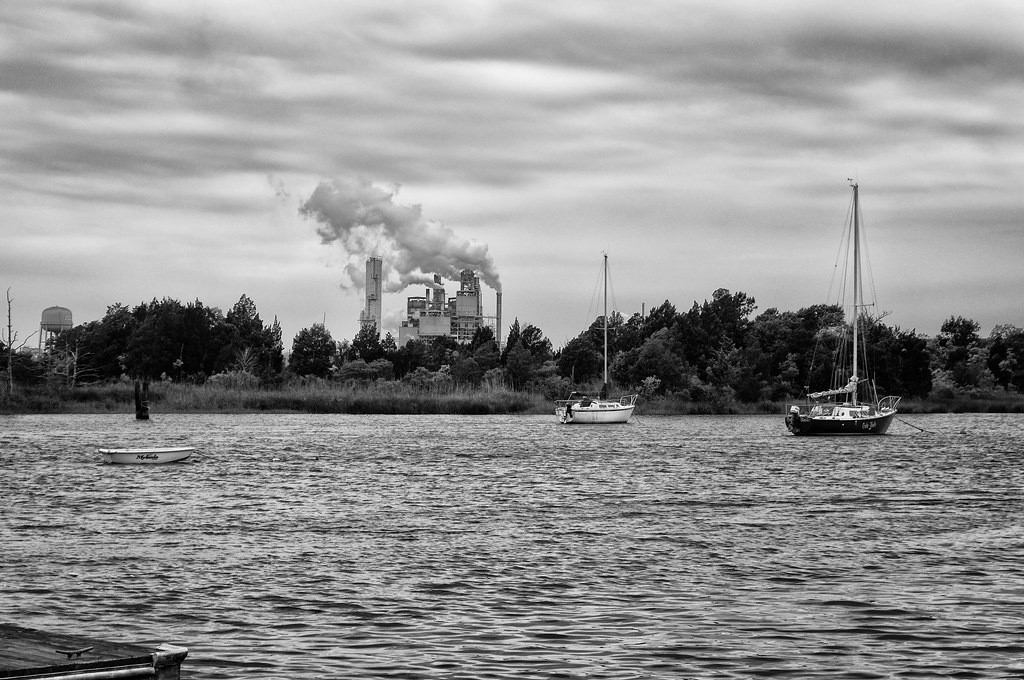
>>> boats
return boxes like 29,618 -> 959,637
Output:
100,445 -> 198,464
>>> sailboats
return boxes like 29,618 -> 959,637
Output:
554,252 -> 640,426
784,179 -> 902,436
